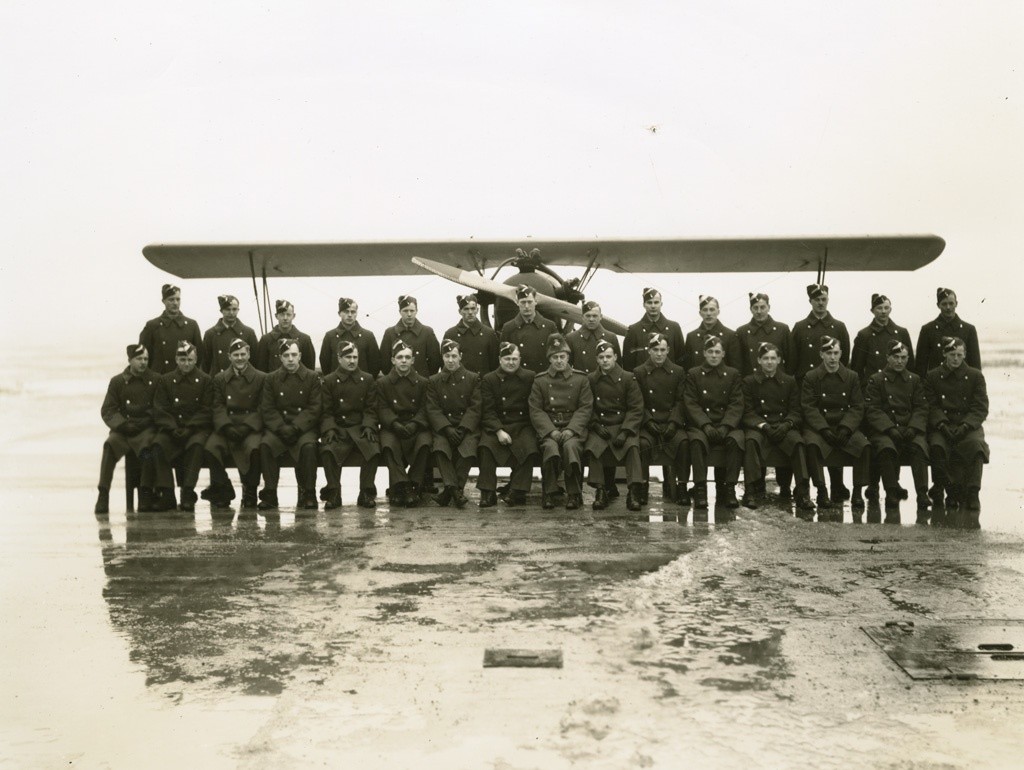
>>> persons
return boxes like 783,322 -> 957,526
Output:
682,295 -> 741,374
851,293 -> 917,390
317,340 -> 381,509
631,331 -> 691,507
150,338 -> 212,511
258,338 -> 323,511
922,335 -> 989,511
319,297 -> 383,379
380,295 -> 441,379
786,283 -> 850,389
585,337 -> 645,512
799,335 -> 872,510
736,293 -> 794,375
528,333 -> 595,509
864,339 -> 932,509
621,287 -> 685,374
94,344 -> 161,517
683,334 -> 746,509
205,337 -> 266,510
425,339 -> 483,508
564,299 -> 623,376
374,338 -> 433,507
202,295 -> 258,378
254,299 -> 317,375
139,284 -> 202,374
441,294 -> 501,377
475,341 -> 540,506
915,287 -> 982,376
742,341 -> 816,511
498,282 -> 561,376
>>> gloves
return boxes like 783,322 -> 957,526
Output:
593,419 -> 970,449
115,418 -> 466,449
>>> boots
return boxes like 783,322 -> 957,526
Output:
94,480 -> 982,513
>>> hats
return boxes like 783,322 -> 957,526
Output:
126,334 -> 963,362
161,281 -> 955,315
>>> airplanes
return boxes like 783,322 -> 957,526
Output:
144,234 -> 946,340
